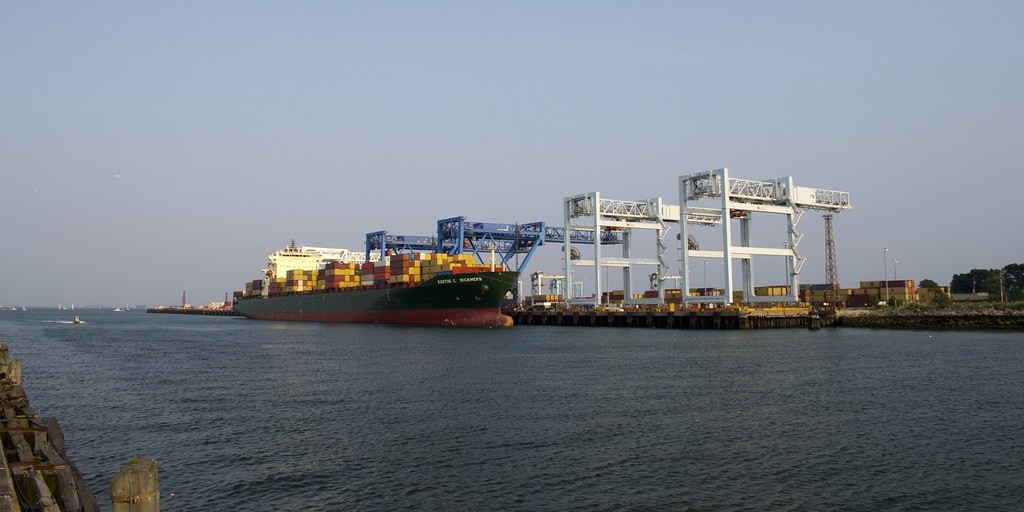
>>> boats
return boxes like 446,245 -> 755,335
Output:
232,238 -> 521,328
74,315 -> 80,324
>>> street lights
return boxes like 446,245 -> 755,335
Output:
883,247 -> 889,301
702,260 -> 708,292
892,259 -> 899,280
